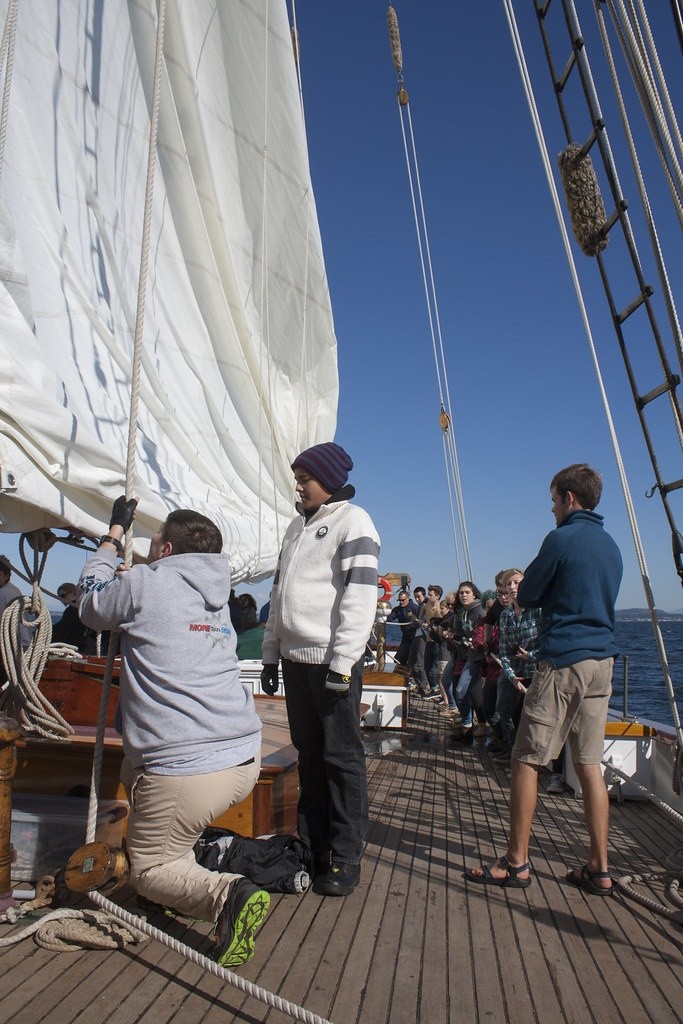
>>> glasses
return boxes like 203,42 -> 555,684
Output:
295,475 -> 314,485
495,588 -> 510,597
61,591 -> 72,598
397,597 -> 408,602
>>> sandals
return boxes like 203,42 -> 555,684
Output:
565,865 -> 613,895
465,856 -> 531,887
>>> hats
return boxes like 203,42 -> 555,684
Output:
290,442 -> 354,495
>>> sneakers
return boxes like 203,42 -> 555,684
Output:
314,861 -> 360,895
136,894 -> 203,921
485,734 -> 507,750
201,874 -> 271,968
472,722 -> 492,736
545,772 -> 564,794
494,738 -> 515,763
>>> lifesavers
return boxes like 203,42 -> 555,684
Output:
378,577 -> 392,600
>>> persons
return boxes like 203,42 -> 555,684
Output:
75,495 -> 270,969
227,588 -> 272,660
50,583 -> 121,656
0,554 -> 35,654
387,568 -> 565,793
260,442 -> 380,897
466,463 -> 623,896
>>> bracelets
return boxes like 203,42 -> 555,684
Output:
100,535 -> 122,552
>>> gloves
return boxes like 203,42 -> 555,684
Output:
324,669 -> 351,702
261,664 -> 279,696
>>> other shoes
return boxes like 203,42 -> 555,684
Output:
435,701 -> 449,708
412,689 -> 431,698
438,704 -> 449,711
459,724 -> 473,738
448,713 -> 461,721
439,706 -> 459,716
422,690 -> 441,700
